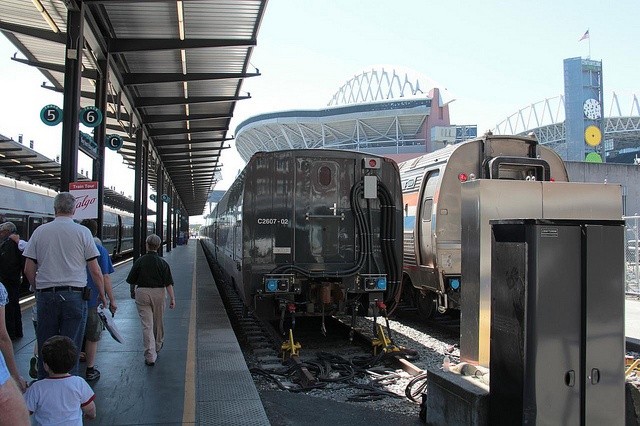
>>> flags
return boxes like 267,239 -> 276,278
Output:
578,28 -> 590,41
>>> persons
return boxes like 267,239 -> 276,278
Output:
0,281 -> 30,426
0,349 -> 30,426
0,220 -> 23,342
79,219 -> 118,379
23,335 -> 96,426
21,193 -> 107,380
125,234 -> 175,367
8,234 -> 39,378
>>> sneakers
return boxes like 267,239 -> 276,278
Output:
86,367 -> 100,381
145,359 -> 155,366
29,357 -> 40,380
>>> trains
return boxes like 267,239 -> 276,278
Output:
0,174 -> 173,288
395,130 -> 569,319
199,148 -> 404,332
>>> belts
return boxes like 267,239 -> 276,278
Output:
35,286 -> 86,292
